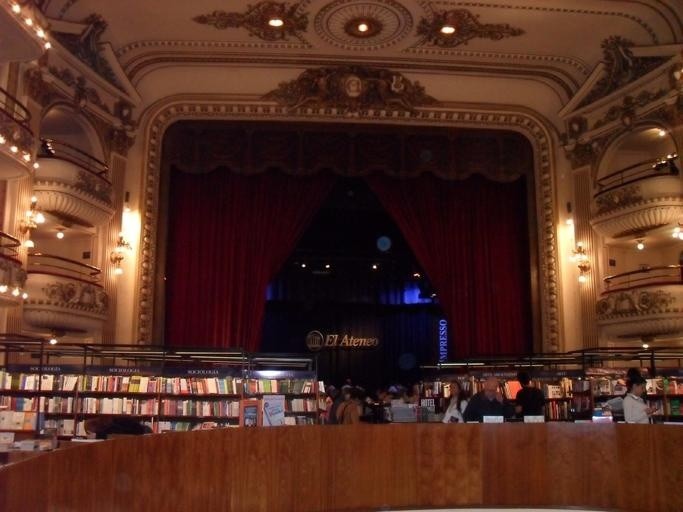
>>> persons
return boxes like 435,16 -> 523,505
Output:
324,377 -> 420,423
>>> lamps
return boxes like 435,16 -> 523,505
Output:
17,209 -> 46,236
108,231 -> 133,277
570,241 -> 592,283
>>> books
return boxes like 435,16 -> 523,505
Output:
2,432 -> 53,453
244,378 -> 324,426
71,439 -> 104,442
1,371 -> 159,436
424,369 -> 682,423
158,378 -> 241,433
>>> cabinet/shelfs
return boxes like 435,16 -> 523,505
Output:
0,339 -> 320,454
417,347 -> 682,423
